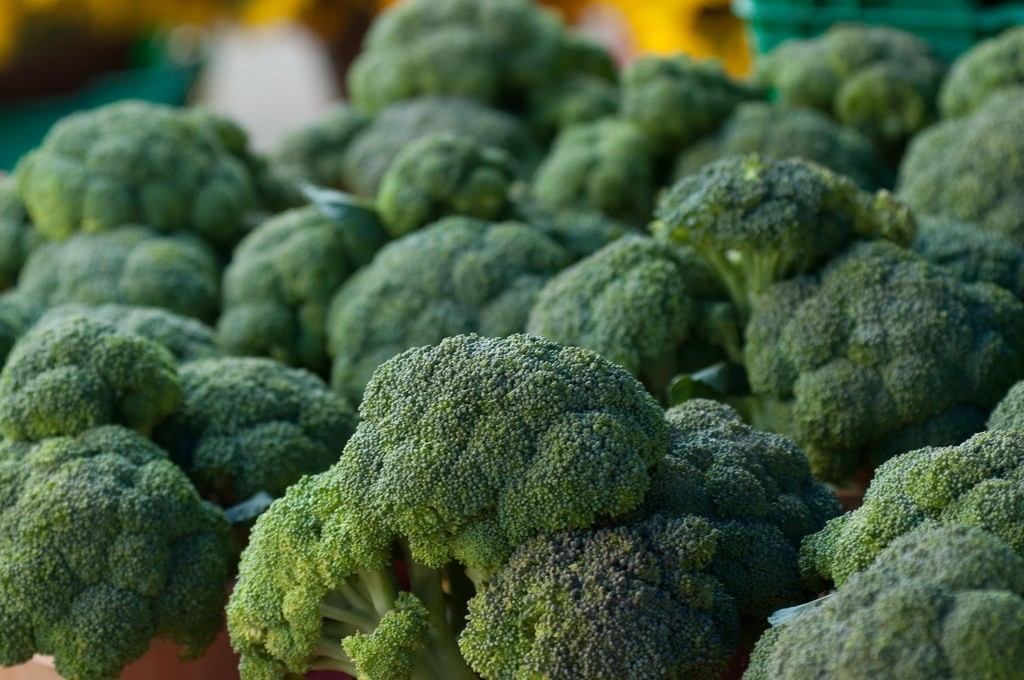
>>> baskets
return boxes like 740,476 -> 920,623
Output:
730,0 -> 1024,102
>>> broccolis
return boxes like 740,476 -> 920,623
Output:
0,0 -> 1024,680
